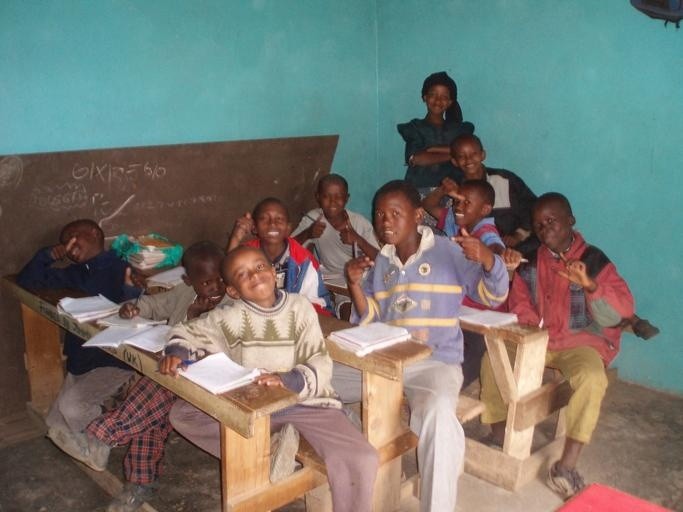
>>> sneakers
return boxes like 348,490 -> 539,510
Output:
44,422 -> 110,473
270,424 -> 301,484
99,482 -> 158,512
543,459 -> 587,498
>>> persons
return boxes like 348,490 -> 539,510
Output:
345,133 -> 665,510
87,239 -> 377,512
15,220 -> 145,375
396,70 -> 474,227
230,174 -> 379,322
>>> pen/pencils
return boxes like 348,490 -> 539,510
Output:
352,240 -> 358,258
302,211 -> 314,223
133,288 -> 146,310
519,259 -> 528,263
155,360 -> 194,373
66,236 -> 79,250
